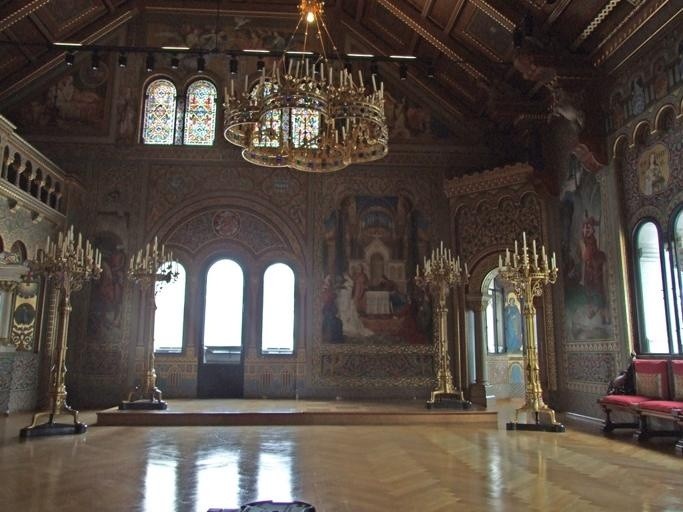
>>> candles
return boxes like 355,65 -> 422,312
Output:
126,234 -> 178,273
410,239 -> 469,281
37,223 -> 103,270
495,230 -> 559,276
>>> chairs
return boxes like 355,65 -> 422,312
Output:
592,347 -> 668,448
635,351 -> 682,450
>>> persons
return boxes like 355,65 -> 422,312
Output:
322,262 -> 420,342
574,210 -> 608,324
644,150 -> 665,195
504,298 -> 521,352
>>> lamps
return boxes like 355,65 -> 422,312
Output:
62,43 -> 437,81
220,0 -> 390,177
